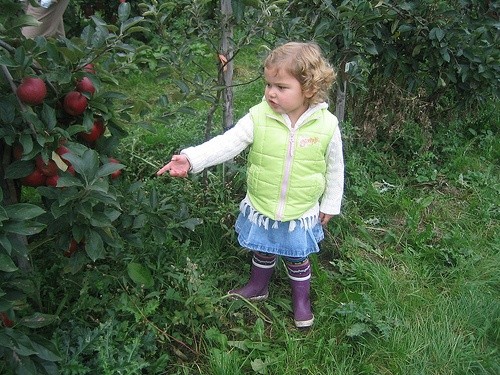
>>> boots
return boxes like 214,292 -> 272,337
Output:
282,259 -> 314,327
227,252 -> 276,301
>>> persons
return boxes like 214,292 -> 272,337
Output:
157,42 -> 344,327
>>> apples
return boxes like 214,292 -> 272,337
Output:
0,312 -> 13,328
19,63 -> 122,187
68,240 -> 77,252
